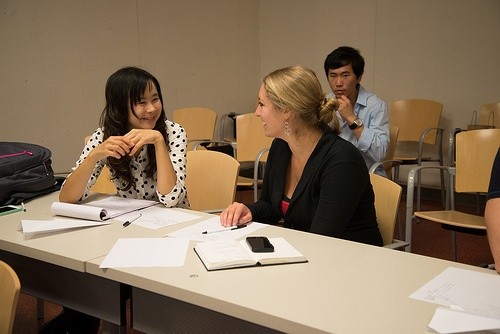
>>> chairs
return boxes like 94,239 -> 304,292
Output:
87,98 -> 500,263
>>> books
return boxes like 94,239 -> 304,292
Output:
51,193 -> 159,222
194,237 -> 308,272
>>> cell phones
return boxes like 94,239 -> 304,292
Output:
246,236 -> 274,252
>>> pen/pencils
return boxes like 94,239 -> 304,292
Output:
122,214 -> 142,226
21,202 -> 26,212
202,224 -> 247,234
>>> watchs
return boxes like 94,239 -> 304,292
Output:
348,117 -> 363,130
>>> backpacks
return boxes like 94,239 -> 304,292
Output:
0,141 -> 67,214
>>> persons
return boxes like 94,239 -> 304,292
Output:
484,146 -> 500,274
324,47 -> 391,178
59,66 -> 192,210
221,65 -> 383,247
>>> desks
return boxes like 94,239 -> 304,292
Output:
0,189 -> 500,334
